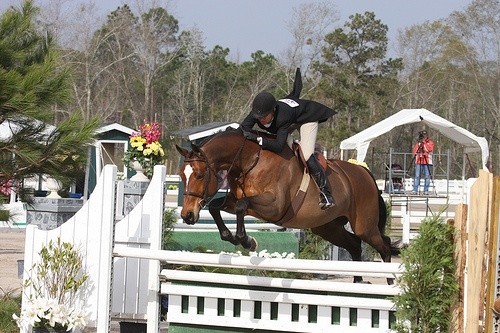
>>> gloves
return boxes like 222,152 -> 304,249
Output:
243,131 -> 258,143
226,126 -> 237,131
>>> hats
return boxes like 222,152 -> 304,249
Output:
250,90 -> 275,118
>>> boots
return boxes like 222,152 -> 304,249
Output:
306,153 -> 337,207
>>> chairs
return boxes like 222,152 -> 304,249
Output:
384,163 -> 406,195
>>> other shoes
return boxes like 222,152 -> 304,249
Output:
423,190 -> 429,195
410,191 -> 418,194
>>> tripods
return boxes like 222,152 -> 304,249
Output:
398,142 -> 437,196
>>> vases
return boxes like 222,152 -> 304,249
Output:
130,157 -> 152,181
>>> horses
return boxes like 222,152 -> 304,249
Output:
176,127 -> 401,285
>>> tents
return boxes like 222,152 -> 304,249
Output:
340,109 -> 490,174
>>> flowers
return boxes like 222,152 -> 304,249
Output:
11,296 -> 91,333
121,122 -> 166,177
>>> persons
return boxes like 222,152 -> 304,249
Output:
410,130 -> 434,195
237,68 -> 338,210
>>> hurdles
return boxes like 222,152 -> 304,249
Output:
20,163 -> 500,333
